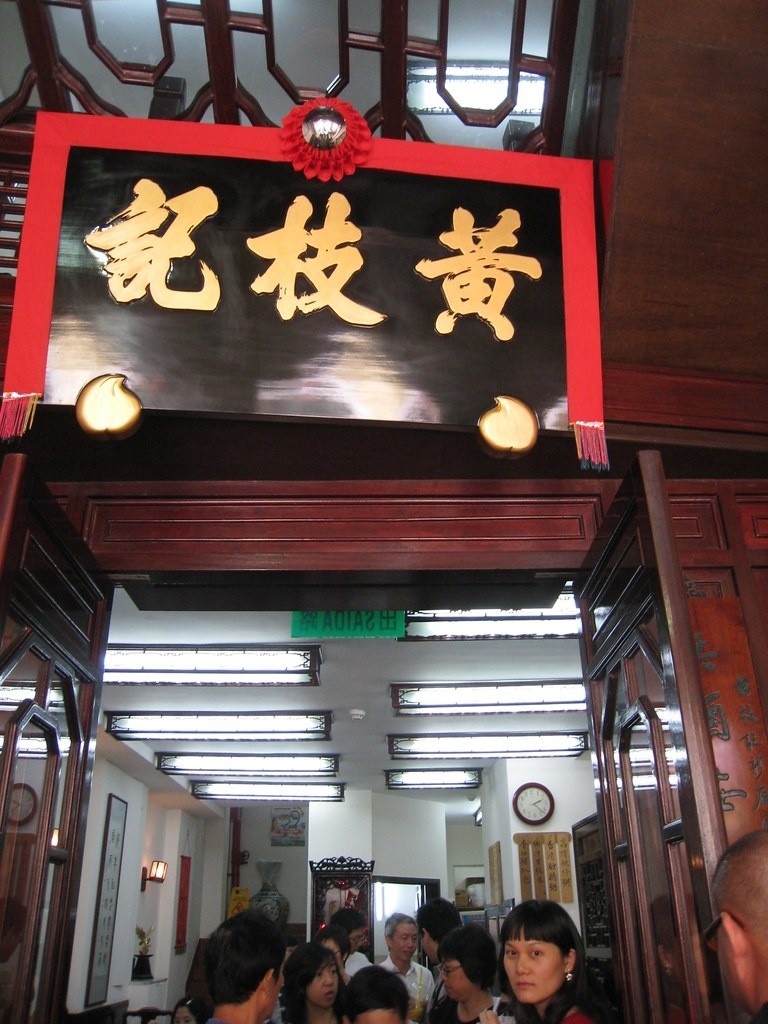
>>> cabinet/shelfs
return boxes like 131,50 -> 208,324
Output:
308,856 -> 376,962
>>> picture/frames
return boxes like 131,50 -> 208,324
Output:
84,793 -> 129,1008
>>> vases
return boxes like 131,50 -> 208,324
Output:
248,860 -> 289,934
132,955 -> 154,980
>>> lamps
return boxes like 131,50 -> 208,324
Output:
155,751 -> 340,777
0,731 -> 70,760
0,679 -> 65,715
190,779 -> 346,803
389,680 -> 587,717
384,767 -> 484,789
386,731 -> 590,760
103,643 -> 323,687
103,710 -> 336,742
141,860 -> 168,892
396,585 -> 579,642
473,806 -> 483,827
593,702 -> 680,792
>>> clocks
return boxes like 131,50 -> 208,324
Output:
513,782 -> 554,825
8,783 -> 37,826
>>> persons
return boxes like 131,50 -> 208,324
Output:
378,912 -> 435,1002
166,898 -> 595,1024
711,827 -> 768,1024
330,908 -> 373,976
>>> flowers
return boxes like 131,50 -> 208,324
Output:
136,926 -> 156,955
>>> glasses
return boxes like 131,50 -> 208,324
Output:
348,934 -> 366,944
702,912 -> 745,965
434,964 -> 461,977
